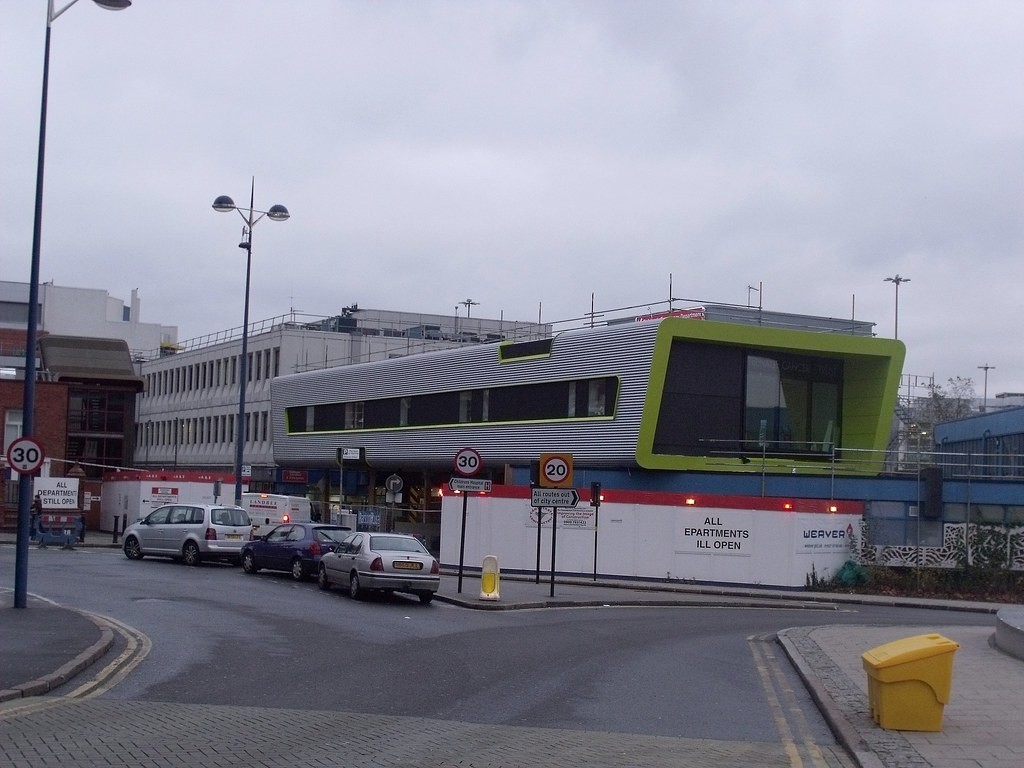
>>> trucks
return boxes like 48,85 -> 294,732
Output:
238,492 -> 311,534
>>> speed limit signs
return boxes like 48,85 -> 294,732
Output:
539,452 -> 574,488
7,437 -> 45,475
454,447 -> 481,477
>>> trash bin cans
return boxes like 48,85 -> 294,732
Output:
861,633 -> 960,732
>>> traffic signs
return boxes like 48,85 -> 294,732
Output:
449,478 -> 492,494
530,488 -> 579,508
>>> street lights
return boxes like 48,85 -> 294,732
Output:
212,175 -> 293,511
457,299 -> 481,321
976,364 -> 996,419
10,1 -> 136,602
883,273 -> 911,340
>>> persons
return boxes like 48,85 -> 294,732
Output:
30,495 -> 45,540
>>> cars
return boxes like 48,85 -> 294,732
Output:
318,532 -> 440,604
122,503 -> 252,567
239,522 -> 355,582
